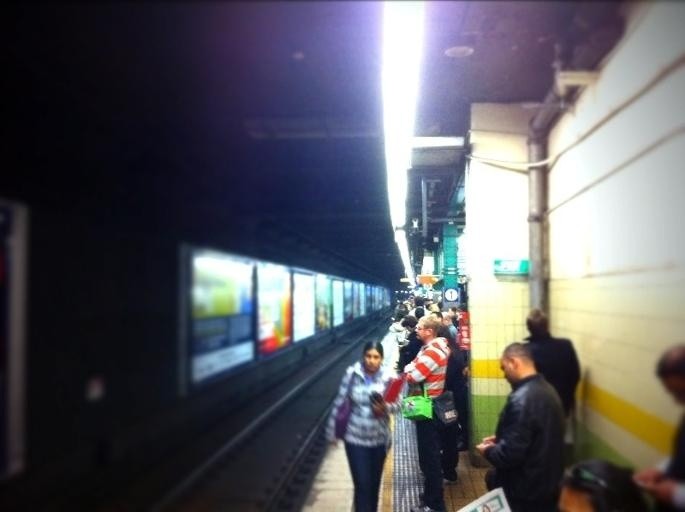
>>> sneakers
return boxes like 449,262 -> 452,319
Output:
410,493 -> 445,512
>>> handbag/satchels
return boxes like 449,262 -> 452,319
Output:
335,395 -> 352,438
401,391 -> 458,426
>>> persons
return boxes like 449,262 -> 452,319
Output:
326,340 -> 402,511
395,293 -> 468,512
520,308 -> 580,481
631,342 -> 685,511
476,343 -> 567,511
